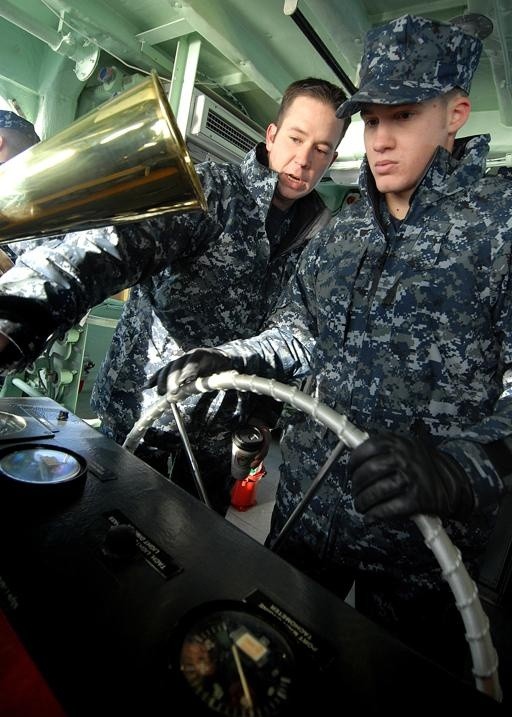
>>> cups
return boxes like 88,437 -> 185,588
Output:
231,426 -> 263,479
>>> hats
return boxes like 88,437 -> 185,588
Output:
0,106 -> 42,146
335,14 -> 484,120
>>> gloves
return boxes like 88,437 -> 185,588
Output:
345,430 -> 475,528
138,346 -> 233,398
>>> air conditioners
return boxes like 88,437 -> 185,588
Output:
189,91 -> 263,170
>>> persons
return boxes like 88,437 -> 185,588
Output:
0,111 -> 66,275
0,79 -> 351,519
142,14 -> 512,665
